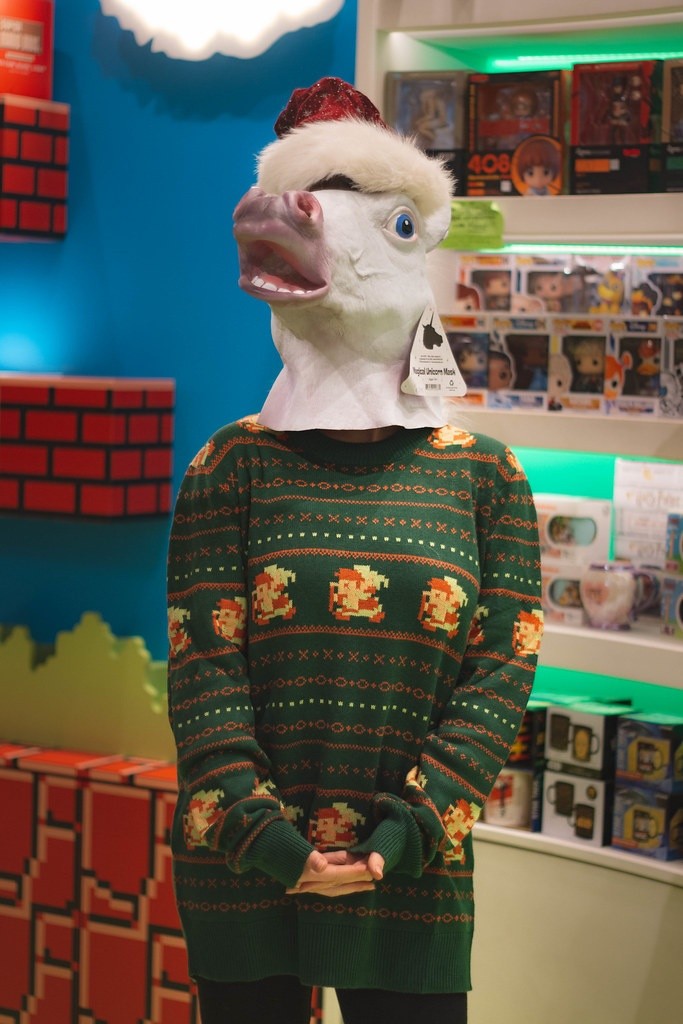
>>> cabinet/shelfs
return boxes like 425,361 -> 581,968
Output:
321,1 -> 683,1024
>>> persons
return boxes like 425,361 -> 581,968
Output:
448,270 -> 683,397
165,77 -> 543,1024
419,80 -> 634,144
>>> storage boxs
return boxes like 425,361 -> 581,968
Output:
385,57 -> 683,196
541,703 -> 683,862
439,252 -> 683,423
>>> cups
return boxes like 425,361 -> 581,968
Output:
581,561 -> 657,633
549,713 -> 575,750
637,739 -> 662,774
632,808 -> 660,844
546,782 -> 574,815
572,724 -> 599,762
566,803 -> 596,840
